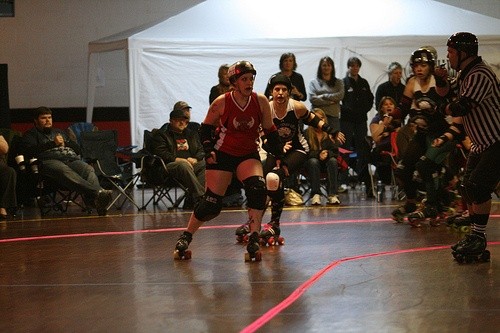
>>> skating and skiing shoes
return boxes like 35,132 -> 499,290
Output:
173,231 -> 192,260
390,193 -> 427,223
450,231 -> 491,265
442,198 -> 472,233
408,193 -> 440,227
245,231 -> 262,263
235,222 -> 261,242
260,224 -> 284,247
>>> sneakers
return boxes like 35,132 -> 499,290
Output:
311,194 -> 322,204
338,183 -> 349,193
326,195 -> 341,205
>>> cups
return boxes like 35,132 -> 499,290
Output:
30,158 -> 39,173
15,155 -> 25,170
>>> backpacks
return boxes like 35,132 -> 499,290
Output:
283,188 -> 305,207
223,188 -> 243,206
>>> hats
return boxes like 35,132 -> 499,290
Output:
174,102 -> 191,110
170,110 -> 188,119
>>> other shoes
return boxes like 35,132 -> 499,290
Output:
0,213 -> 17,221
95,190 -> 113,216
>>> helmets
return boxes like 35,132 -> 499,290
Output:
447,32 -> 478,56
410,49 -> 435,66
271,74 -> 291,90
228,63 -> 256,84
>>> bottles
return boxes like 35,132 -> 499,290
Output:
377,181 -> 383,202
360,182 -> 366,200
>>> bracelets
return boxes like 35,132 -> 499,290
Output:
434,76 -> 447,88
438,135 -> 449,143
438,103 -> 449,115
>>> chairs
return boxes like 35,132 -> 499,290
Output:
14,137 -> 92,218
96,129 -> 192,216
294,161 -> 428,201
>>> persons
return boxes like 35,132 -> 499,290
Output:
13,106 -> 113,216
174,46 -> 406,204
175,61 -> 292,253
235,72 -> 346,238
303,108 -> 341,205
434,32 -> 500,255
151,109 -> 206,209
0,128 -> 18,220
383,45 -> 500,233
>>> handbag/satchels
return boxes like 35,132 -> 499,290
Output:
143,155 -> 161,185
